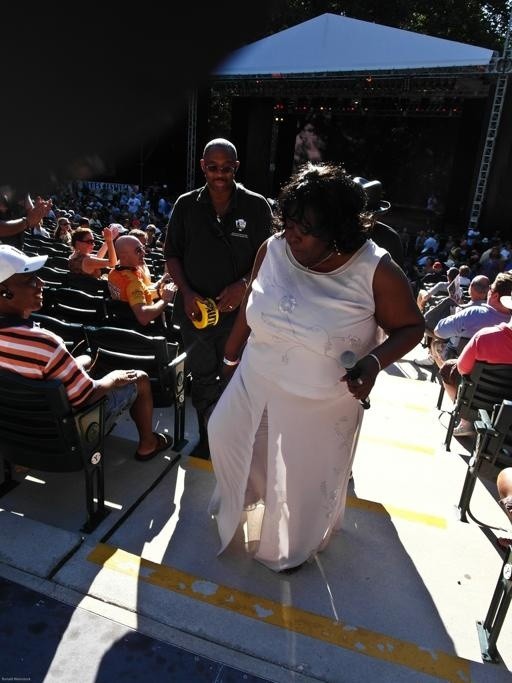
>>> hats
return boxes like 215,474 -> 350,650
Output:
443,259 -> 456,269
352,176 -> 392,216
107,223 -> 129,233
0,244 -> 49,283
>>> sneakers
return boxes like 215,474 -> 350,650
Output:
453,422 -> 475,437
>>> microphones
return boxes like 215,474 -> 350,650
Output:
339,350 -> 371,410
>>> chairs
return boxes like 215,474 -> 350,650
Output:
1,217 -> 186,452
0,375 -> 118,536
477,524 -> 512,654
458,399 -> 511,523
422,282 -> 511,452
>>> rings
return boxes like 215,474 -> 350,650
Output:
191,312 -> 195,316
228,305 -> 233,309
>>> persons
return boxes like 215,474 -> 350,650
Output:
163,137 -> 271,460
205,164 -> 425,575
400,220 -> 512,551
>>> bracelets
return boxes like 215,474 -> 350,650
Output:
367,353 -> 381,372
240,277 -> 249,289
223,355 -> 239,366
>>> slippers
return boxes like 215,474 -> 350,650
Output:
134,432 -> 173,462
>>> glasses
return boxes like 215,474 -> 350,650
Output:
81,239 -> 95,244
205,164 -> 235,174
135,245 -> 146,255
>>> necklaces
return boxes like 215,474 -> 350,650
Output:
304,248 -> 336,270
211,188 -> 232,223
